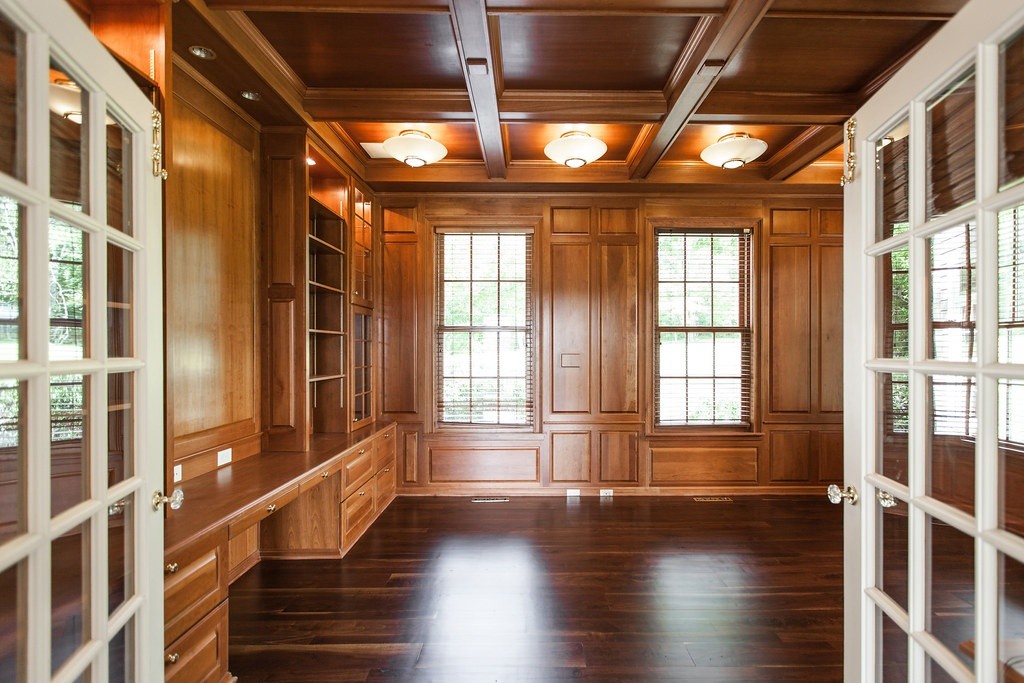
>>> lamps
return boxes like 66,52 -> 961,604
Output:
382,129 -> 448,168
543,131 -> 607,168
700,131 -> 768,169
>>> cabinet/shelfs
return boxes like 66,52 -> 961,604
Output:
164,181 -> 842,683
